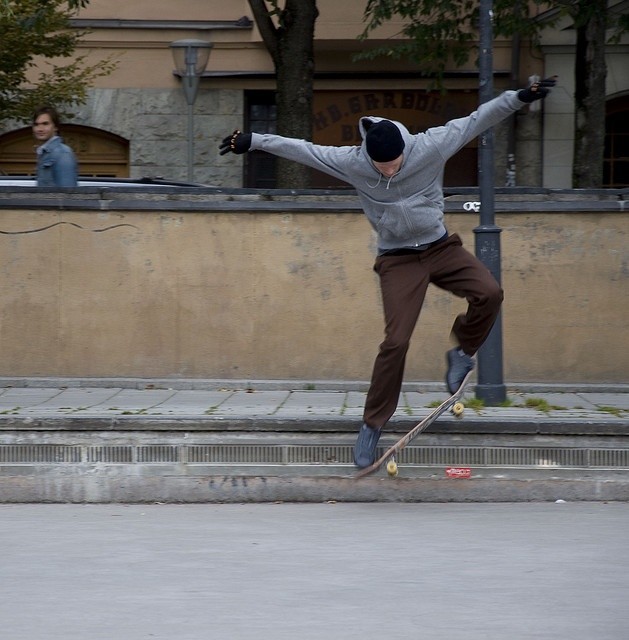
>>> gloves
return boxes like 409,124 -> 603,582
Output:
219,129 -> 251,156
518,75 -> 558,103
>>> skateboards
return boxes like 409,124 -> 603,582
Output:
352,370 -> 474,483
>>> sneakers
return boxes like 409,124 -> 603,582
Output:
353,422 -> 382,468
446,346 -> 476,395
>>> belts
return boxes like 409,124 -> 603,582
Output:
378,231 -> 448,257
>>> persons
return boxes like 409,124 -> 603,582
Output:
218,73 -> 558,469
31,103 -> 78,188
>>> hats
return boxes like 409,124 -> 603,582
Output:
366,120 -> 405,162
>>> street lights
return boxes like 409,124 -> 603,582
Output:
170,39 -> 214,183
